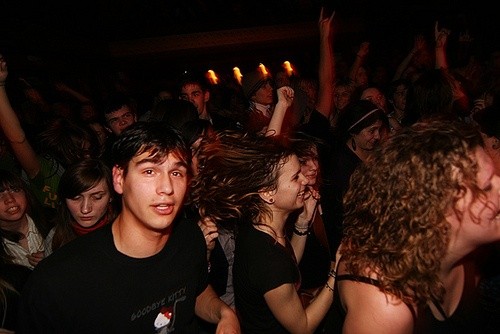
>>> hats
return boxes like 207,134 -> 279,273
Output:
241,68 -> 273,99
340,100 -> 386,137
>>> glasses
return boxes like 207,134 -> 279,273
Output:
366,94 -> 384,104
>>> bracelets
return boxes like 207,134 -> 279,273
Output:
328,269 -> 337,279
325,282 -> 335,293
294,223 -> 309,229
292,228 -> 311,236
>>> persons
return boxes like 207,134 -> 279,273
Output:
242,69 -> 278,135
321,99 -> 389,261
189,128 -> 344,334
276,131 -> 329,280
99,97 -> 138,138
330,118 -> 500,334
0,162 -> 56,283
271,68 -> 301,131
52,159 -> 118,252
179,73 -> 210,119
157,86 -> 175,104
0,54 -> 108,209
13,120 -> 241,334
282,7 -> 500,139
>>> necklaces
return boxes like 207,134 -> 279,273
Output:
254,219 -> 287,238
